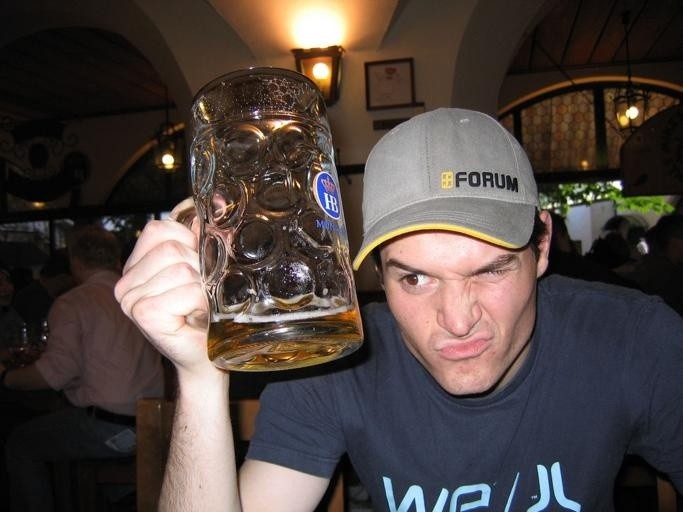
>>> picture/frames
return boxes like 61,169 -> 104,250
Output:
363,56 -> 416,111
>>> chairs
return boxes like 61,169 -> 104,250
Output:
131,398 -> 345,511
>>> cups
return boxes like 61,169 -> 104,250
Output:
166,64 -> 366,371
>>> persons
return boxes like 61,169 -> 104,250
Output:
550,209 -> 683,317
115,107 -> 683,512
0,256 -> 70,348
0,234 -> 179,512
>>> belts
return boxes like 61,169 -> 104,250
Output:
88,406 -> 136,427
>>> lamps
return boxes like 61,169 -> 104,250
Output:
289,45 -> 342,106
611,19 -> 650,134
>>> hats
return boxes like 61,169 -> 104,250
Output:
351,107 -> 542,271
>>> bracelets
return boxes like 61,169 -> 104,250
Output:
0,369 -> 8,385
